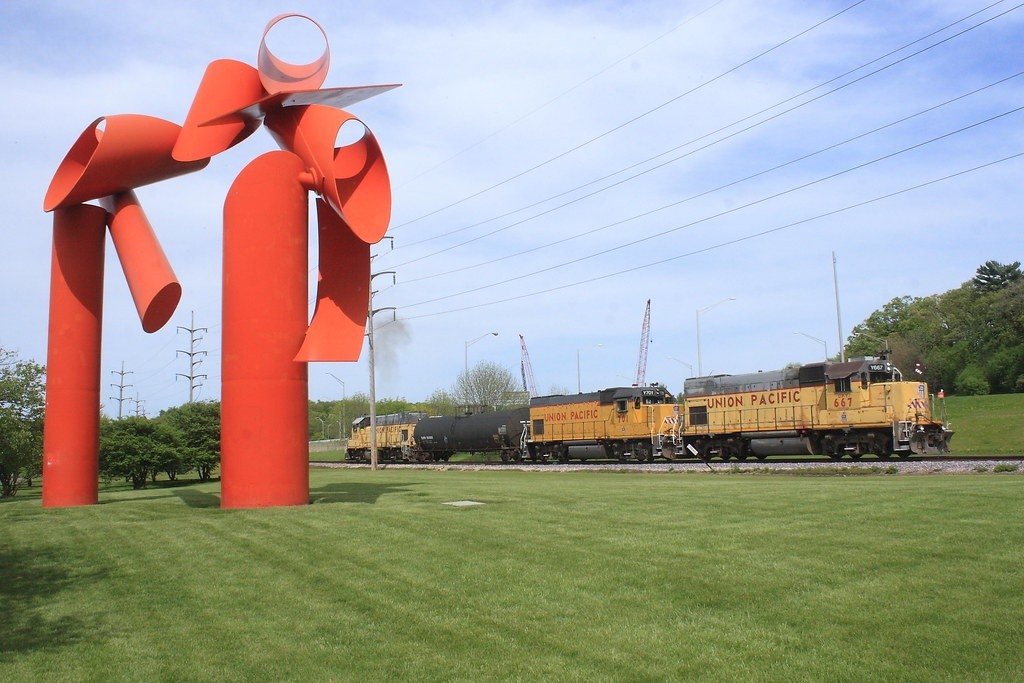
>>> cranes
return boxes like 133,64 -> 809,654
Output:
517,334 -> 538,399
637,299 -> 654,387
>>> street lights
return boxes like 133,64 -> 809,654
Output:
793,331 -> 828,362
315,416 -> 326,439
577,343 -> 604,394
464,332 -> 499,402
328,420 -> 339,439
697,295 -> 736,377
666,355 -> 693,378
854,332 -> 889,360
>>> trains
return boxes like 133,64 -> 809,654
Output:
344,349 -> 954,465
